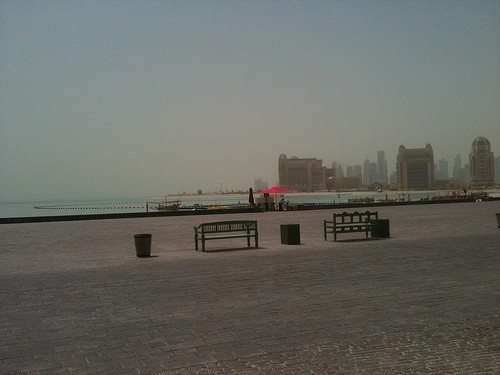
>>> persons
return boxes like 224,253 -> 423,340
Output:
279,198 -> 284,211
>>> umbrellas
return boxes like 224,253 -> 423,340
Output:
259,187 -> 292,202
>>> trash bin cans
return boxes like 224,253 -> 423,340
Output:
496,213 -> 500,228
134,233 -> 152,257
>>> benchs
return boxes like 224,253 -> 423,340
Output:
324,210 -> 379,242
194,220 -> 258,252
